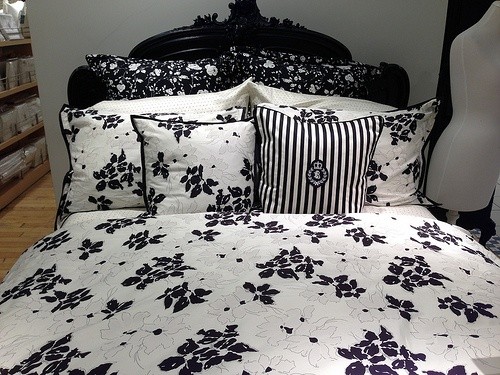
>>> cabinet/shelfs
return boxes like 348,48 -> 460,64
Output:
0,0 -> 51,211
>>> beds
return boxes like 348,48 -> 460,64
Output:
0,0 -> 500,375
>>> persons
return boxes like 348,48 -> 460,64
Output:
426,1 -> 500,212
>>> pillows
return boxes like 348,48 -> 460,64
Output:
56,104 -> 246,216
246,82 -> 399,118
85,76 -> 253,120
253,97 -> 440,206
85,52 -> 243,100
228,39 -> 383,99
129,114 -> 259,216
253,104 -> 385,214
